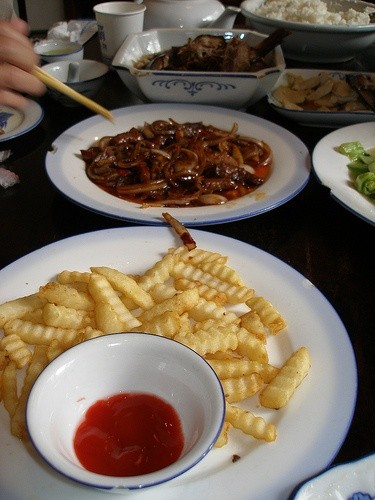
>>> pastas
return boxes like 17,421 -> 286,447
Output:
87,118 -> 260,208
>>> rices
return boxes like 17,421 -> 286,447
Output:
251,0 -> 375,26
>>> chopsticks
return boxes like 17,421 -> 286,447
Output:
33,64 -> 115,124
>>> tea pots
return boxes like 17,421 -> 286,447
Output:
133,0 -> 241,30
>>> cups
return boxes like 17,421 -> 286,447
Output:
33,42 -> 84,61
93,1 -> 146,70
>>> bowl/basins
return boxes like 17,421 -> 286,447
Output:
37,60 -> 110,108
25,331 -> 227,493
110,27 -> 286,112
240,0 -> 375,63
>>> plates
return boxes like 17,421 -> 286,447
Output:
44,103 -> 311,226
0,97 -> 45,144
267,69 -> 375,128
0,226 -> 357,500
311,122 -> 375,226
293,453 -> 375,500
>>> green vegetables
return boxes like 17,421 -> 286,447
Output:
335,141 -> 375,194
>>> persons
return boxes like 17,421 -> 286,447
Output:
0,0 -> 45,111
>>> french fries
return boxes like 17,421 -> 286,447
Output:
271,71 -> 369,114
0,212 -> 310,448
